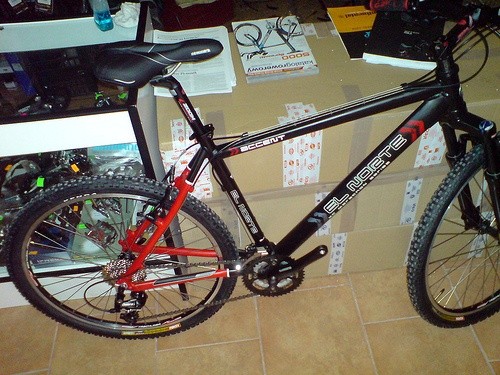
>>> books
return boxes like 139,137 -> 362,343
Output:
362,10 -> 445,71
231,15 -> 320,84
327,5 -> 377,61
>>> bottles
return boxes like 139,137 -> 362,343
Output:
89,0 -> 114,32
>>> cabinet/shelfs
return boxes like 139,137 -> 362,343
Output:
0,0 -> 189,309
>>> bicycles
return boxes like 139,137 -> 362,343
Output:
4,0 -> 500,340
233,15 -> 304,60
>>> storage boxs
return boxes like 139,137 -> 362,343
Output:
155,17 -> 500,283
